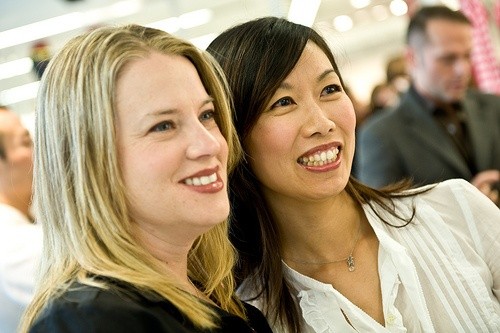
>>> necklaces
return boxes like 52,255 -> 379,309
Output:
280,204 -> 365,272
184,275 -> 203,299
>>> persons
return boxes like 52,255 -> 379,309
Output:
1,106 -> 61,333
18,24 -> 277,333
355,57 -> 412,133
201,18 -> 500,333
354,5 -> 500,210
